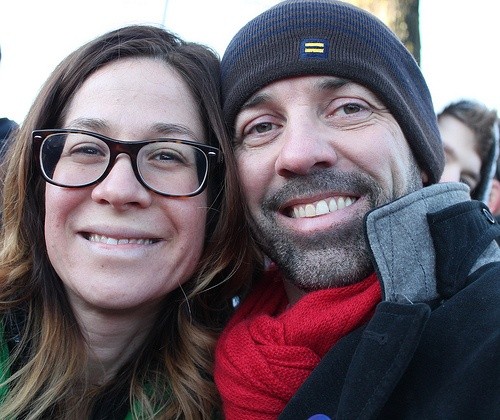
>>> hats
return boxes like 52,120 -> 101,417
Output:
219,0 -> 444,187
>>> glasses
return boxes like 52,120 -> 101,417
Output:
30,128 -> 222,200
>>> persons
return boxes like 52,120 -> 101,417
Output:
434,99 -> 496,205
0,26 -> 265,420
214,1 -> 500,420
